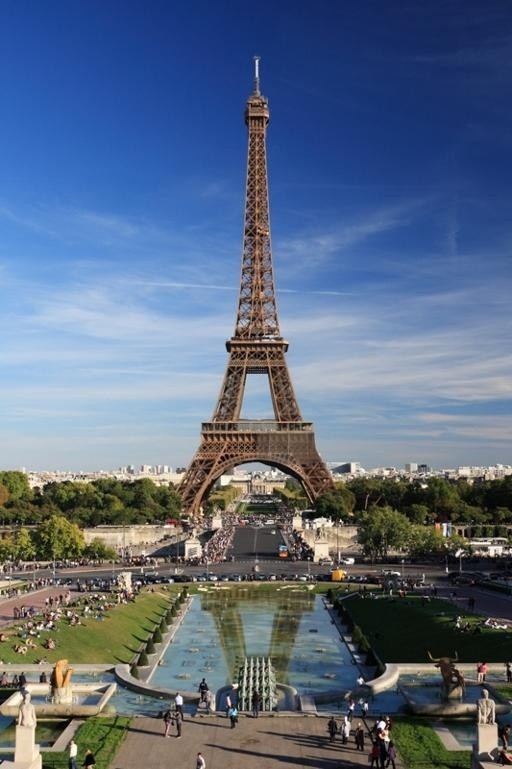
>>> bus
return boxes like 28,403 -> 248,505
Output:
276,543 -> 289,562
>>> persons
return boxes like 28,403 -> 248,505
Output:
196,752 -> 206,769
1,554 -> 142,656
160,678 -> 260,739
476,690 -> 496,725
477,660 -> 512,682
1,672 -> 47,689
499,725 -> 512,751
67,740 -> 96,769
327,698 -> 397,769
119,513 -> 314,568
17,692 -> 37,727
345,572 -> 440,607
449,588 -> 509,635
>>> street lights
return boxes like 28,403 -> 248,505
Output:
306,556 -> 311,573
400,559 -> 405,577
204,556 -> 209,574
110,559 -> 116,576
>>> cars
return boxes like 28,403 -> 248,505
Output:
447,570 -> 511,584
318,557 -> 334,566
79,569 -> 406,585
341,557 -> 355,566
